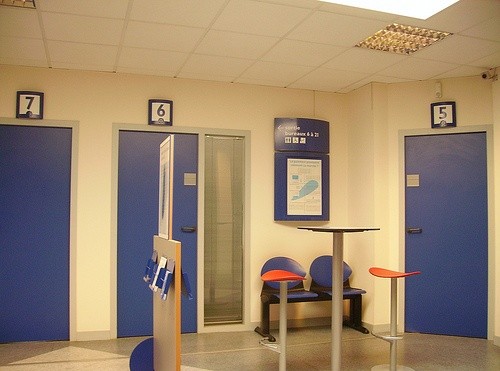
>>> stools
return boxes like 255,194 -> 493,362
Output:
369,264 -> 420,370
258,271 -> 306,371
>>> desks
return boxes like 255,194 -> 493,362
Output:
296,226 -> 381,371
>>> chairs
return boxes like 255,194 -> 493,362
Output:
255,254 -> 368,341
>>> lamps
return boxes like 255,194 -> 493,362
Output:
355,21 -> 456,55
